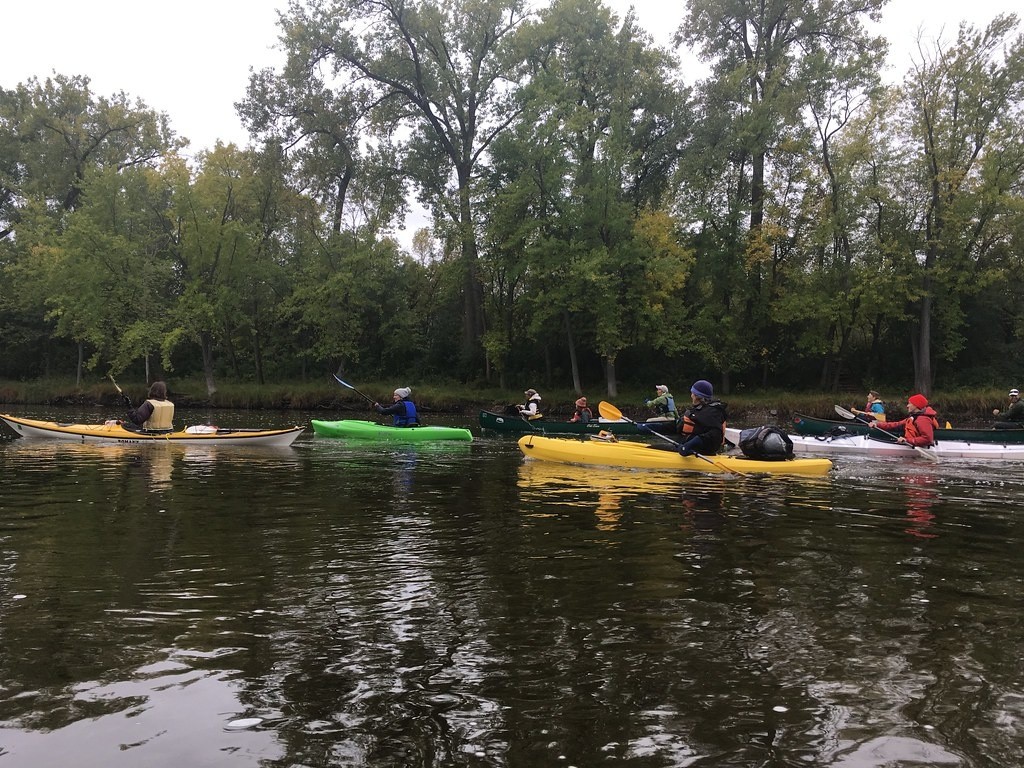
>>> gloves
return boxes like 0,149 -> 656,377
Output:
637,423 -> 662,433
644,398 -> 649,403
678,436 -> 703,456
122,395 -> 131,406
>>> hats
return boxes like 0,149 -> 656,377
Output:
909,394 -> 929,410
525,389 -> 536,395
690,380 -> 713,398
575,397 -> 586,407
1008,388 -> 1019,396
394,386 -> 411,398
655,385 -> 668,392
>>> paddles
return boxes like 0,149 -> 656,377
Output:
598,400 -> 746,476
516,405 -> 535,429
332,374 -> 372,403
109,374 -> 138,413
834,404 -> 947,461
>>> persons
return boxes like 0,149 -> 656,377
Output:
514,389 -> 543,421
565,397 -> 592,423
869,395 -> 934,445
374,387 -> 421,427
994,388 -> 1024,429
637,380 -> 729,457
123,382 -> 174,434
851,391 -> 885,422
644,385 -> 678,423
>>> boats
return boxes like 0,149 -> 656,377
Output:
791,412 -> 1024,443
0,413 -> 307,446
479,409 -> 684,435
518,435 -> 833,475
725,428 -> 1024,459
310,419 -> 473,441
